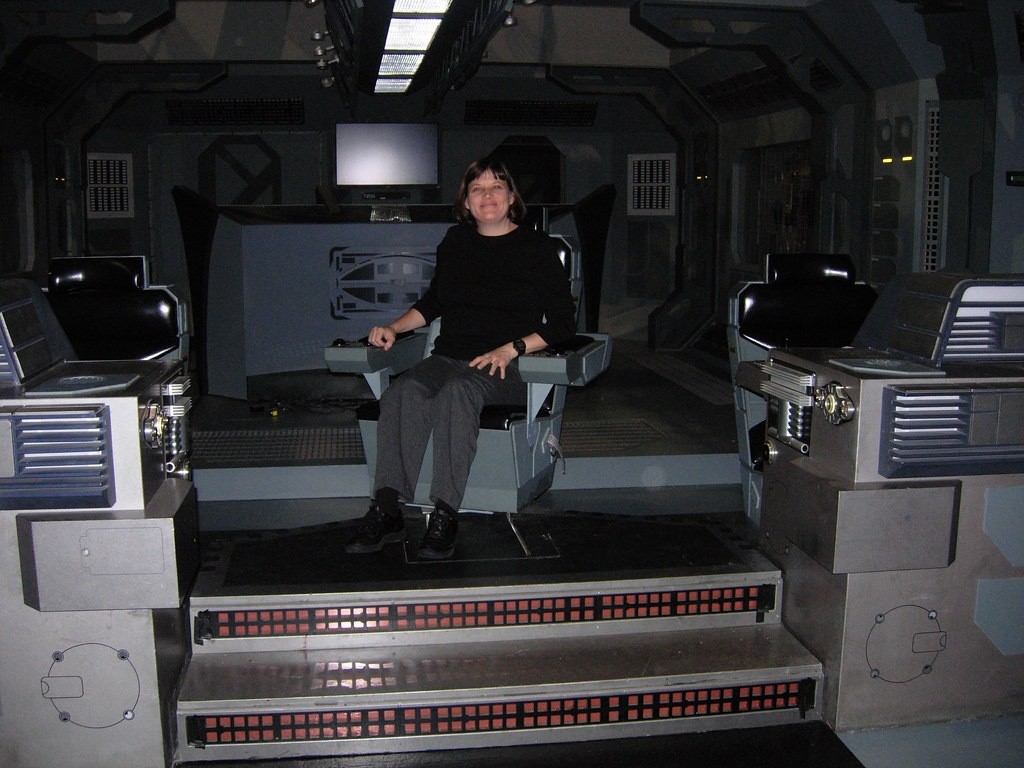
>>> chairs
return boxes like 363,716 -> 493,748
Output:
324,232 -> 614,513
47,256 -> 189,406
728,254 -> 877,541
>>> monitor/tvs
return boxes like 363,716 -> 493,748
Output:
332,120 -> 442,189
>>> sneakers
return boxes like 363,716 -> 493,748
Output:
344,504 -> 407,553
417,509 -> 459,559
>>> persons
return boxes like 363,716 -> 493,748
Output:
343,157 -> 576,559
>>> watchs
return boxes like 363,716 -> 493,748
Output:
512,337 -> 526,357
